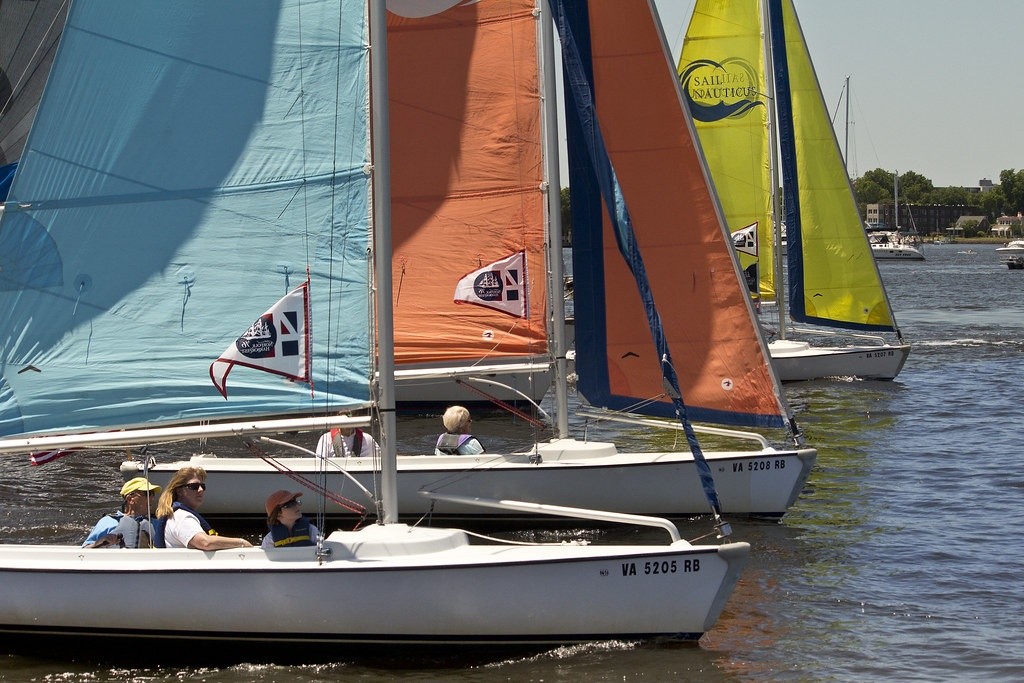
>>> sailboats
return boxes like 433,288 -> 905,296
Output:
0,1 -> 927,652
776,71 -> 926,256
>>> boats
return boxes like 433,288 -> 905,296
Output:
995,239 -> 1024,270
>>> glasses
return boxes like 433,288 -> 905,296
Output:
280,499 -> 301,508
175,483 -> 206,491
130,490 -> 155,497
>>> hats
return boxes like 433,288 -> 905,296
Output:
265,489 -> 304,517
120,477 -> 162,498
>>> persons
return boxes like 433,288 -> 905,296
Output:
80,476 -> 162,550
434,405 -> 487,455
260,490 -> 324,550
315,410 -> 382,457
155,467 -> 254,551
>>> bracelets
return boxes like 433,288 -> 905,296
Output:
239,538 -> 244,548
